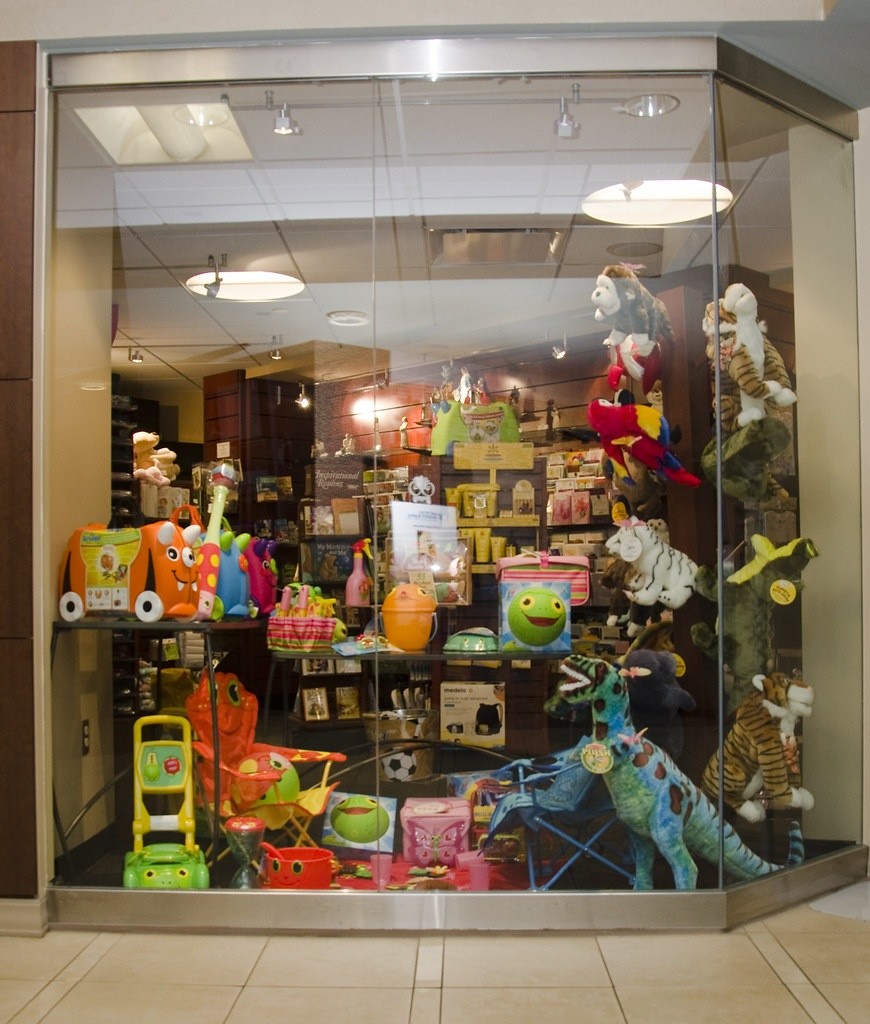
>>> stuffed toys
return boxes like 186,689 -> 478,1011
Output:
690,534 -> 819,719
587,381 -> 701,487
608,333 -> 662,396
622,650 -> 695,763
605,515 -> 699,608
701,417 -> 791,504
558,653 -> 805,890
648,518 -> 669,546
601,559 -> 666,636
591,265 -> 673,344
616,621 -> 674,666
698,672 -> 815,823
702,283 -> 797,498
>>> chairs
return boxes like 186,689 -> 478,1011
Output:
189,671 -> 346,876
490,734 -> 638,890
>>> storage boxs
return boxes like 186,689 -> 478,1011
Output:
439,680 -> 507,752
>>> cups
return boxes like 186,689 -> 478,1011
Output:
455,849 -> 484,869
371,854 -> 392,886
468,863 -> 490,891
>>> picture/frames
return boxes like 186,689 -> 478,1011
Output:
337,659 -> 363,676
334,686 -> 363,721
302,688 -> 330,721
303,658 -> 335,676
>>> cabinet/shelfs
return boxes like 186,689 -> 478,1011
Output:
459,516 -> 543,574
113,395 -> 142,718
544,522 -> 626,612
203,368 -> 315,536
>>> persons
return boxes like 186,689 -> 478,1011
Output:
458,367 -> 492,405
551,406 -> 560,429
343,433 -> 355,454
399,416 -> 409,448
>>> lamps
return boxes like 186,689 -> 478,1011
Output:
551,342 -> 566,357
127,348 -> 145,363
296,380 -> 307,403
269,349 -> 283,361
551,84 -> 585,139
206,252 -> 228,299
271,103 -> 295,133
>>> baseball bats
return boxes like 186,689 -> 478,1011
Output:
196,484 -> 229,620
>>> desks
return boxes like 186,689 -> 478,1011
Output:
83,617 -> 268,887
280,648 -> 569,803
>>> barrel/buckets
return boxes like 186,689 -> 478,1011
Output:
362,710 -> 438,782
379,585 -> 437,650
263,848 -> 334,889
